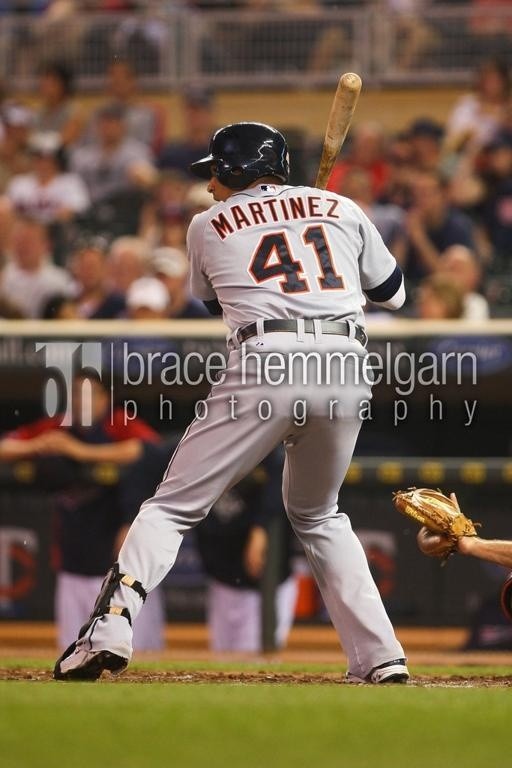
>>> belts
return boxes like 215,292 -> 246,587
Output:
228,319 -> 367,348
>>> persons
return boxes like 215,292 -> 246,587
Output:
195,447 -> 309,655
49,118 -> 414,684
1,365 -> 170,654
388,485 -> 512,623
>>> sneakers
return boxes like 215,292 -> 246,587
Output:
53,640 -> 129,682
344,659 -> 410,684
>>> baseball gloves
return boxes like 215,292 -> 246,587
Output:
392,486 -> 482,568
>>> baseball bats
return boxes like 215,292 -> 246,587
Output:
315,73 -> 362,191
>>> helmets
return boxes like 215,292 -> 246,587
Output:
190,122 -> 291,190
